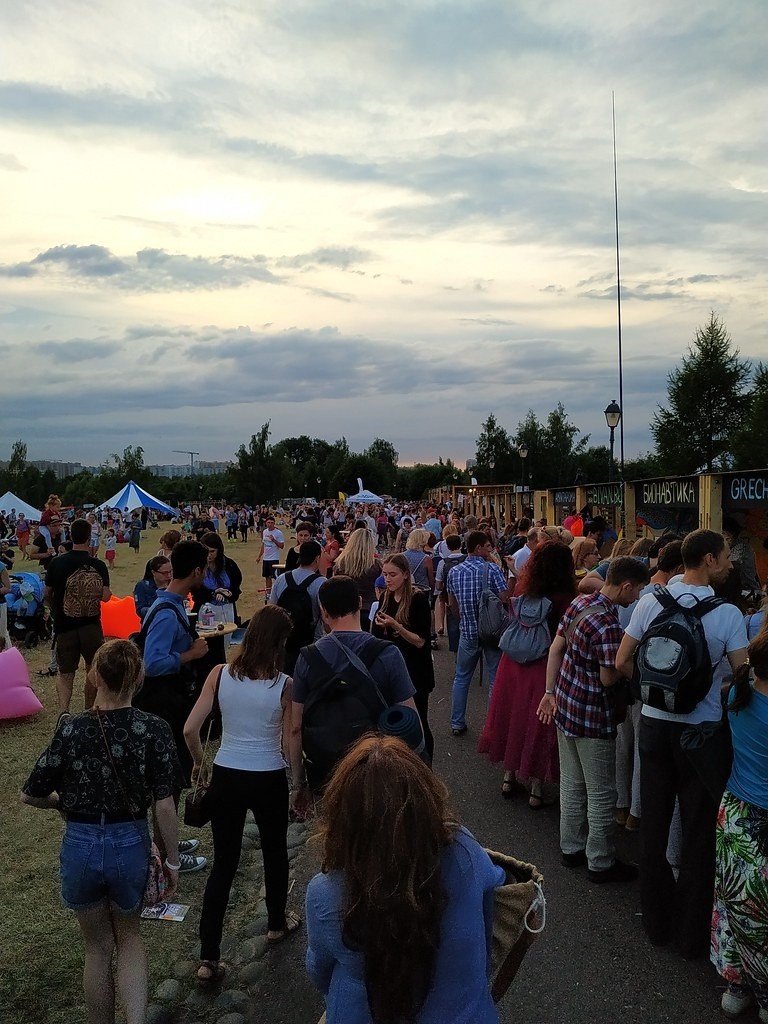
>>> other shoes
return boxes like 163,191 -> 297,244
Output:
721,988 -> 768,1024
430,626 -> 444,651
452,724 -> 468,735
616,809 -> 640,832
647,932 -> 700,959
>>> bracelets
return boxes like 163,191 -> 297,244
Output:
165,858 -> 181,870
396,624 -> 403,633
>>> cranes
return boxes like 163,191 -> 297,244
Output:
172,450 -> 199,473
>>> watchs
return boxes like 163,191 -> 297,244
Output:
546,689 -> 554,694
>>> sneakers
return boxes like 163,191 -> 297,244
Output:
54,712 -> 72,736
560,851 -> 640,883
175,838 -> 208,874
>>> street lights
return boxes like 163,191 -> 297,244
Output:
468,468 -> 473,486
453,473 -> 458,485
517,439 -> 529,491
489,460 -> 495,485
603,400 -> 621,482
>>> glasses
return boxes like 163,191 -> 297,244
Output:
153,570 -> 172,577
539,526 -> 553,540
588,551 -> 600,556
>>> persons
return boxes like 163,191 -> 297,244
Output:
19,640 -> 179,1024
615,528 -> 751,945
44,520 -> 112,734
139,539 -> 210,873
183,605 -> 294,988
289,577 -> 426,816
0,561 -> 12,653
448,515 -> 767,884
710,630 -> 768,1024
304,735 -> 506,1024
0,493 -> 468,771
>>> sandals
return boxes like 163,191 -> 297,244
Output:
266,910 -> 301,944
502,779 -> 558,810
35,668 -> 58,676
196,959 -> 226,986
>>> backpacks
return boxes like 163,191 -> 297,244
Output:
430,551 -> 443,578
631,587 -> 726,715
442,554 -> 468,603
476,562 -> 553,663
59,554 -> 105,618
276,570 -> 323,648
410,553 -> 427,583
5,514 -> 13,524
301,639 -> 394,797
129,603 -> 207,742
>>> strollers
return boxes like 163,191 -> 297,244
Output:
5,572 -> 53,648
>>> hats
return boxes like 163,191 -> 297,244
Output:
374,576 -> 387,589
50,515 -> 62,524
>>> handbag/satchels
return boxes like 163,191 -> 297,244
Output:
198,600 -> 234,650
143,839 -> 170,908
184,784 -> 212,828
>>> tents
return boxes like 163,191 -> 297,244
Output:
0,491 -> 42,522
92,480 -> 177,516
347,490 -> 383,507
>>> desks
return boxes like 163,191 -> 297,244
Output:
195,622 -> 238,666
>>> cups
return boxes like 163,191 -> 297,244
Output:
187,613 -> 197,630
